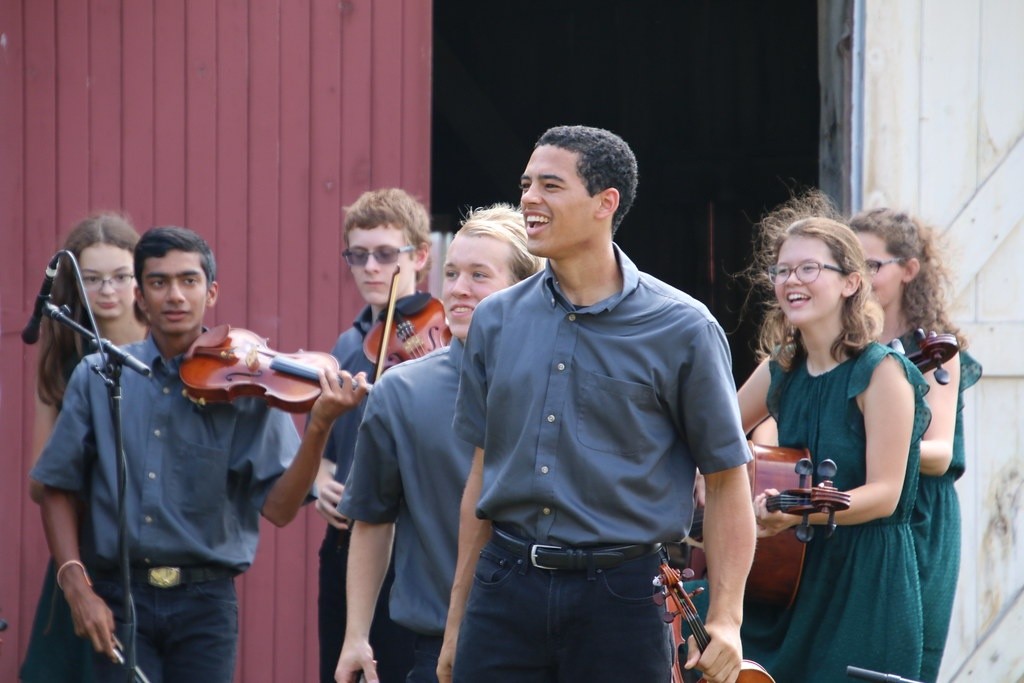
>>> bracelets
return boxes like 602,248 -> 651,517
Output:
57,561 -> 92,590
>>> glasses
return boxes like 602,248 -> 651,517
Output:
865,257 -> 904,273
767,262 -> 842,285
342,245 -> 416,265
76,274 -> 135,290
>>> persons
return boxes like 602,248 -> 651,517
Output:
301,126 -> 981,683
19,211 -> 368,683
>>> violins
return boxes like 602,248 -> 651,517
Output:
362,292 -> 452,371
181,323 -> 373,415
649,562 -> 776,683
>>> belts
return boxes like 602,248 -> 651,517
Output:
491,526 -> 662,570
96,556 -> 233,588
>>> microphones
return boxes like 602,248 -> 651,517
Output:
22,254 -> 59,345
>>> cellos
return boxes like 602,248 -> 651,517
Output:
688,439 -> 851,609
886,324 -> 959,385
